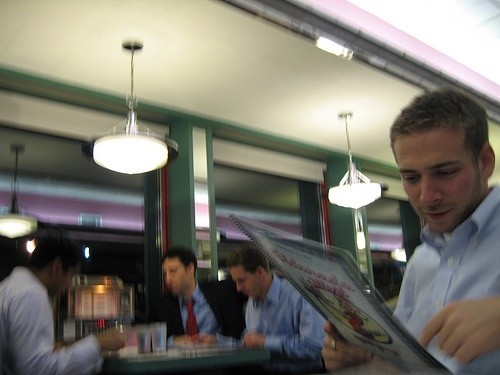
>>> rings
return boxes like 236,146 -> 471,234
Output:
331,339 -> 336,350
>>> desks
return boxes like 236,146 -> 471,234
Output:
97,348 -> 270,375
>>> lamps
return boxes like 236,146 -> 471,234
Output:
322,111 -> 389,208
1,142 -> 40,239
83,40 -> 180,175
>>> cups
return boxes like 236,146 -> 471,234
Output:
135,325 -> 152,354
151,323 -> 166,353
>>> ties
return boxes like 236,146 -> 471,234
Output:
184,300 -> 198,335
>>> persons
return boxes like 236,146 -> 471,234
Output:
0,235 -> 125,375
227,242 -> 327,375
134,244 -> 249,349
322,89 -> 500,375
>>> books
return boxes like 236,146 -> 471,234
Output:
228,213 -> 453,375
